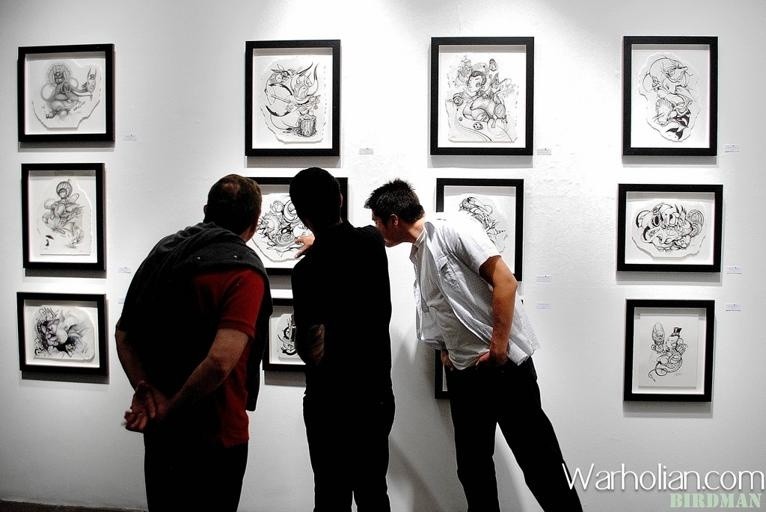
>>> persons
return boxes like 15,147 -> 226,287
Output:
116,174 -> 272,511
289,166 -> 396,511
363,179 -> 586,511
